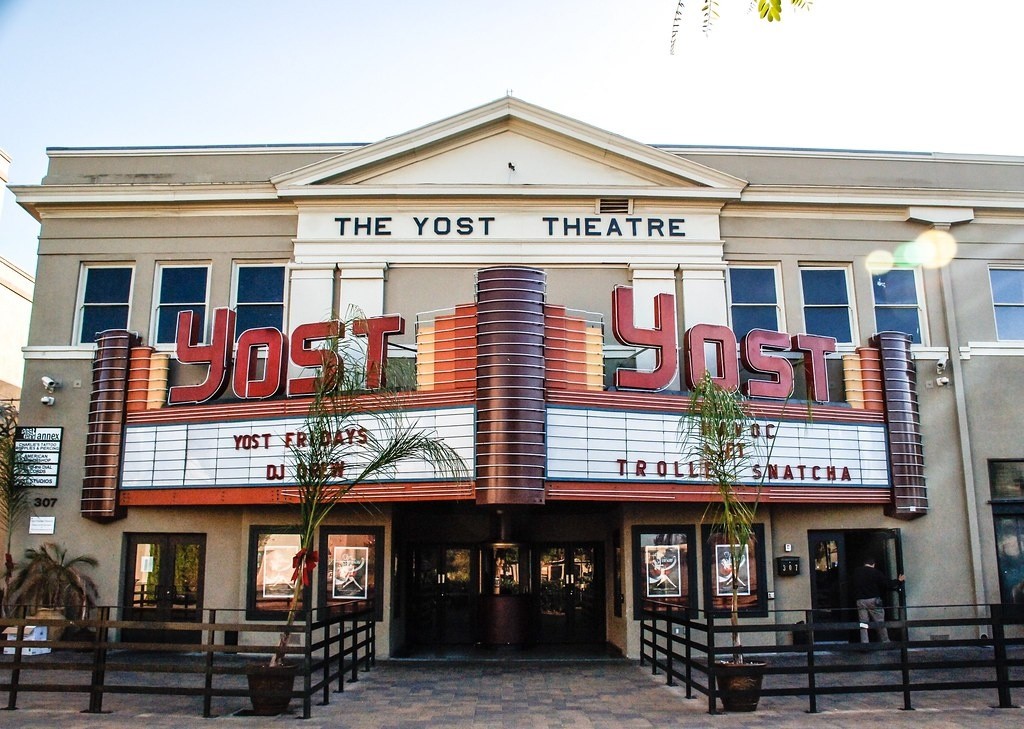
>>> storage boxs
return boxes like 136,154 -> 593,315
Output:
1,626 -> 48,655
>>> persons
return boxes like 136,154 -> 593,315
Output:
853,556 -> 906,651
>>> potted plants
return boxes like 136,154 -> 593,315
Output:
4,542 -> 101,641
674,359 -> 814,713
242,300 -> 473,716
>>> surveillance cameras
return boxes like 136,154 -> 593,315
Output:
42,376 -> 54,393
41,397 -> 55,405
936,377 -> 949,386
936,356 -> 947,374
